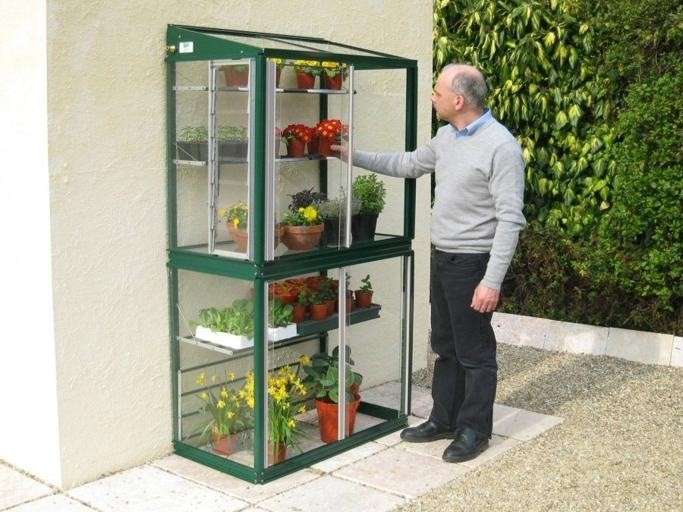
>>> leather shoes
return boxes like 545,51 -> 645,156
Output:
442,428 -> 489,463
400,417 -> 458,443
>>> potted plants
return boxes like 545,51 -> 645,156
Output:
177,125 -> 249,161
352,173 -> 387,243
313,196 -> 363,248
301,343 -> 362,444
216,64 -> 248,88
194,275 -> 373,350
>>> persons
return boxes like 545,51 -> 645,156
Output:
331,63 -> 527,462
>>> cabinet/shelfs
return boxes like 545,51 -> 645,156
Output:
164,23 -> 419,272
165,250 -> 422,484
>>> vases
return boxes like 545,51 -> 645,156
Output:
267,441 -> 287,468
211,425 -> 241,456
296,71 -> 316,89
276,69 -> 281,88
320,71 -> 343,90
276,139 -> 341,158
227,224 -> 285,254
279,223 -> 324,251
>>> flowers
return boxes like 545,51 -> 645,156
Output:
185,367 -> 254,459
321,61 -> 350,82
289,60 -> 322,76
280,204 -> 325,226
218,199 -> 248,230
267,58 -> 286,70
239,349 -> 313,465
276,119 -> 349,143
